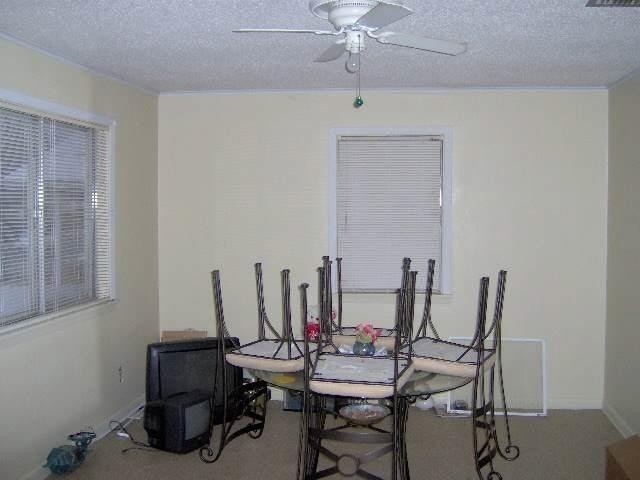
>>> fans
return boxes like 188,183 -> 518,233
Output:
230,1 -> 469,64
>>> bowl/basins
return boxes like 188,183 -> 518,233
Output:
339,403 -> 390,425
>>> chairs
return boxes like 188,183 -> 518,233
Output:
197,253 -> 521,479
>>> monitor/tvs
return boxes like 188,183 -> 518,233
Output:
146,336 -> 243,425
144,392 -> 213,455
